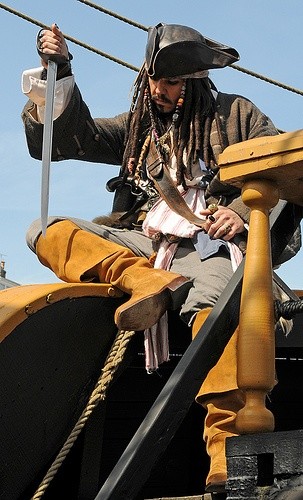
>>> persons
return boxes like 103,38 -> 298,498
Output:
21,23 -> 280,491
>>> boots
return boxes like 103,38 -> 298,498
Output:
36,220 -> 193,331
192,308 -> 245,492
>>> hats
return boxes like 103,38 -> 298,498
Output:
145,22 -> 240,81
